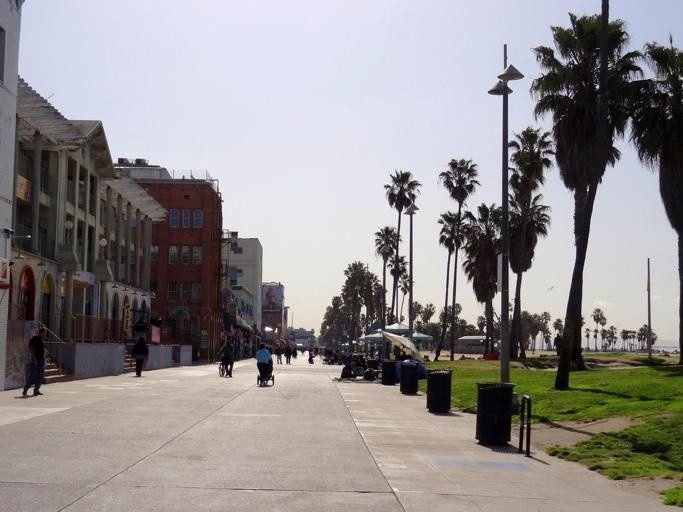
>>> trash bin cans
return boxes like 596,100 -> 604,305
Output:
400,360 -> 421,394
381,361 -> 397,385
475,383 -> 517,445
427,369 -> 453,413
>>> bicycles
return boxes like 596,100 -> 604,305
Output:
215,355 -> 226,376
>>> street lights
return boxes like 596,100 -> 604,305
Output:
487,45 -> 525,380
291,311 -> 295,330
353,280 -> 361,338
403,204 -> 419,342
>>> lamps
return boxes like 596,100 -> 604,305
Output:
13,222 -> 159,299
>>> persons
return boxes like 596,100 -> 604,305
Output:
265,343 -> 341,365
132,336 -> 147,377
217,340 -> 233,378
22,326 -> 46,397
553,333 -> 561,355
264,287 -> 277,310
256,343 -> 272,386
234,342 -> 241,360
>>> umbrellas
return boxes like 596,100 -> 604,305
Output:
340,320 -> 433,359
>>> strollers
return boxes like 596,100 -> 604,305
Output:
256,362 -> 275,387
